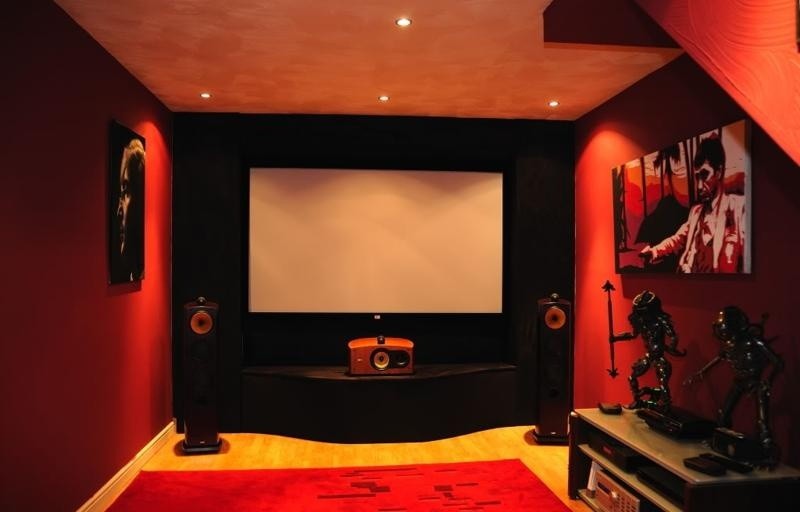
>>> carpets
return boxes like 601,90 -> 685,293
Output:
105,458 -> 572,512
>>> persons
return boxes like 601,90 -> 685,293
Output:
689,300 -> 785,445
641,135 -> 745,274
111,137 -> 145,282
612,289 -> 681,420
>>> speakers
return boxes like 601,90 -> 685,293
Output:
534,294 -> 572,443
347,336 -> 414,375
180,302 -> 218,455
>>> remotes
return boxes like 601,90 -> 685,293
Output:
683,454 -> 726,476
699,453 -> 752,474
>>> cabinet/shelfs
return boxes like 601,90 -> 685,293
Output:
567,409 -> 694,512
